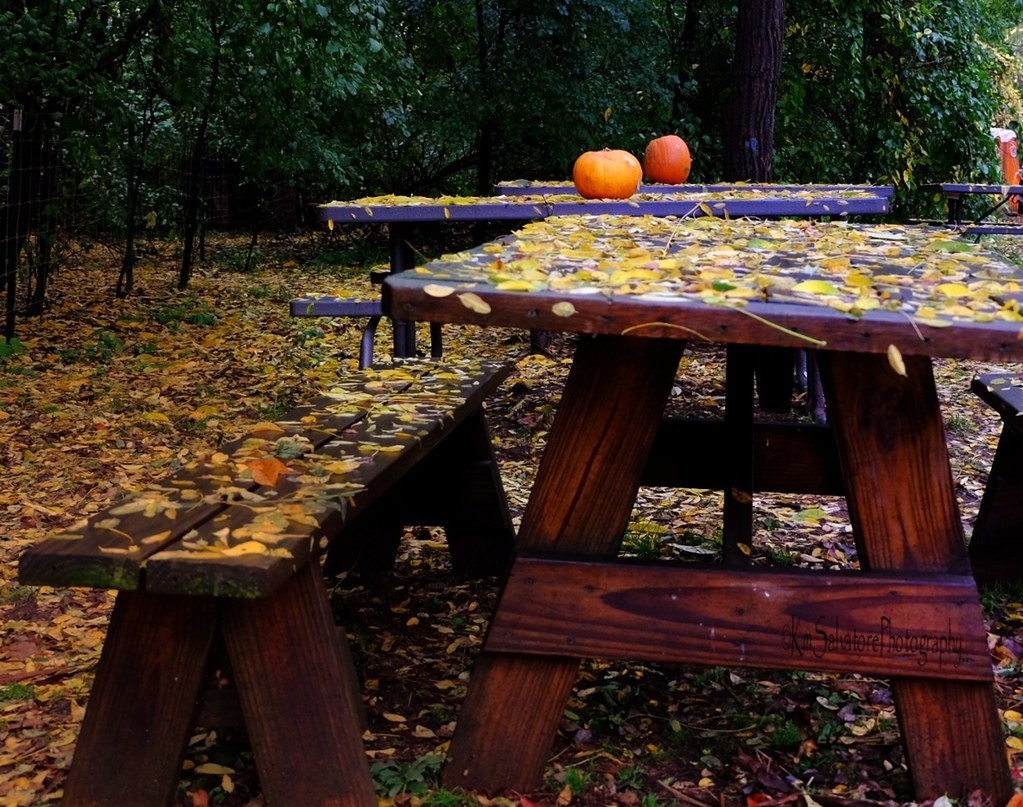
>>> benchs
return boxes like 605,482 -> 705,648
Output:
23,345 -> 524,807
957,367 -> 1016,607
296,288 -> 384,371
928,219 -> 1021,244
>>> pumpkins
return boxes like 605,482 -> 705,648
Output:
573,145 -> 643,200
643,135 -> 690,187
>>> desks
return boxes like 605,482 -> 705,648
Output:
936,182 -> 1021,240
378,209 -> 1018,804
324,165 -> 896,342
